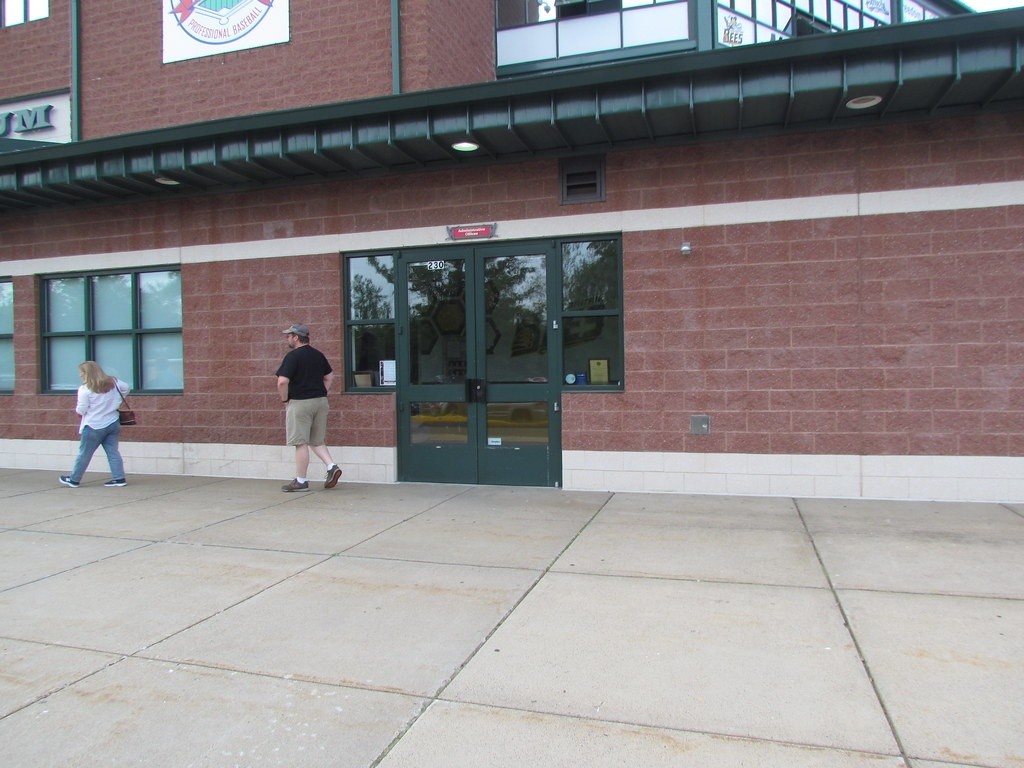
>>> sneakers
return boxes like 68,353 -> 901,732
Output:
104,477 -> 128,487
323,465 -> 342,489
281,477 -> 310,492
59,475 -> 81,487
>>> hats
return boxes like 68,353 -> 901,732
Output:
281,322 -> 310,338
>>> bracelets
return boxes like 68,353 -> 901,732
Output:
282,399 -> 288,403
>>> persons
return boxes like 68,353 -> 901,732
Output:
59,361 -> 130,488
275,324 -> 342,492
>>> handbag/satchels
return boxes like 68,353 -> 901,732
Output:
117,410 -> 136,425
